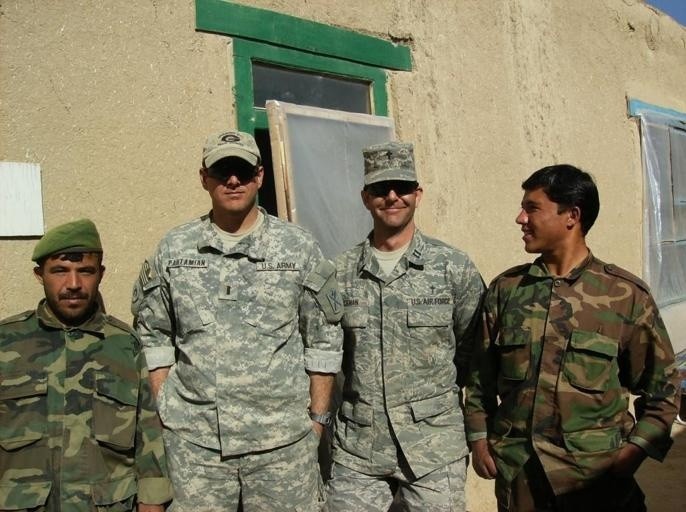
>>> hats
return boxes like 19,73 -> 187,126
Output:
361,141 -> 416,185
201,129 -> 261,170
30,217 -> 103,261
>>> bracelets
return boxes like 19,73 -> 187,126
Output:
309,409 -> 333,428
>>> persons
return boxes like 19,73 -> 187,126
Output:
1,218 -> 172,510
328,142 -> 489,512
463,165 -> 681,510
125,127 -> 344,509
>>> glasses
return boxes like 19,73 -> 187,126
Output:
208,165 -> 257,181
363,183 -> 419,196
